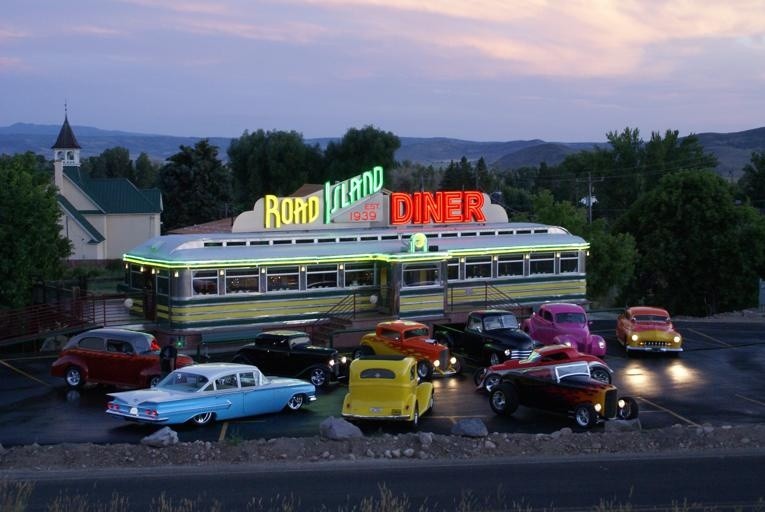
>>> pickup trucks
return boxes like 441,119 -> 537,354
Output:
431,308 -> 545,367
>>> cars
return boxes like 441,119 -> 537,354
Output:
356,319 -> 463,379
231,330 -> 350,389
50,328 -> 194,390
473,343 -> 614,394
519,302 -> 608,357
615,306 -> 686,356
488,361 -> 640,428
339,355 -> 435,427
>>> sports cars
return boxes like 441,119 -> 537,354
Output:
105,364 -> 320,433
